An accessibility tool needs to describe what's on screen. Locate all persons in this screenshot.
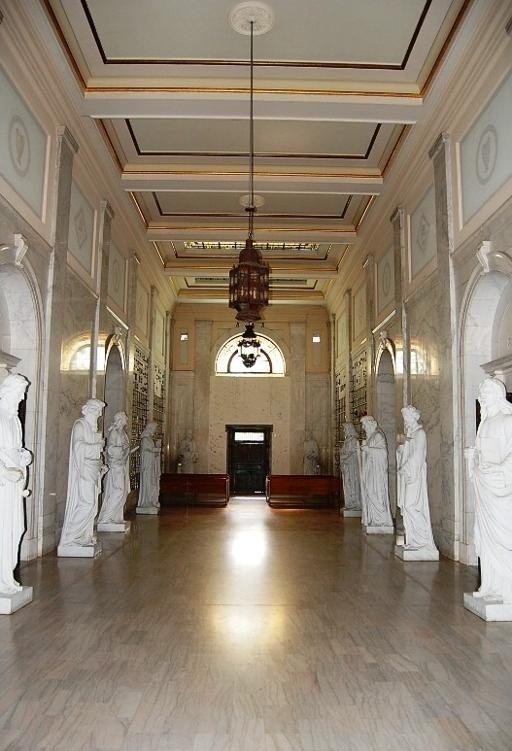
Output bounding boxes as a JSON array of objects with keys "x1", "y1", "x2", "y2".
[
  {"x1": 338, "y1": 422, "x2": 361, "y2": 509},
  {"x1": 356, "y1": 415, "x2": 394, "y2": 527},
  {"x1": 61, "y1": 397, "x2": 109, "y2": 546},
  {"x1": 98, "y1": 410, "x2": 132, "y2": 525},
  {"x1": 299, "y1": 429, "x2": 320, "y2": 475},
  {"x1": 395, "y1": 404, "x2": 434, "y2": 548},
  {"x1": 1, "y1": 374, "x2": 32, "y2": 594},
  {"x1": 137, "y1": 422, "x2": 164, "y2": 509},
  {"x1": 178, "y1": 431, "x2": 198, "y2": 473},
  {"x1": 462, "y1": 377, "x2": 512, "y2": 601}
]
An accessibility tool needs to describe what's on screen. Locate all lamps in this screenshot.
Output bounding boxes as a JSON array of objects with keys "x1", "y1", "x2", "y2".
[{"x1": 228, "y1": 1, "x2": 274, "y2": 369}]
